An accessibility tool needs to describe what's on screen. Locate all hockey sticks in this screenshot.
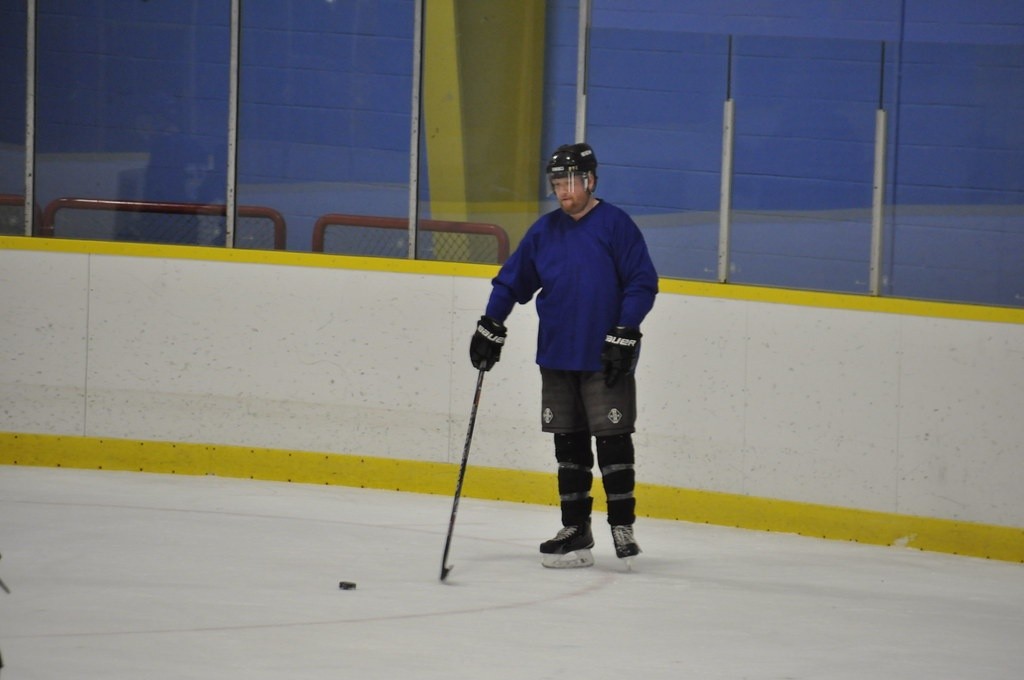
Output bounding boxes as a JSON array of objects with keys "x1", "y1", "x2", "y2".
[{"x1": 440, "y1": 359, "x2": 489, "y2": 581}]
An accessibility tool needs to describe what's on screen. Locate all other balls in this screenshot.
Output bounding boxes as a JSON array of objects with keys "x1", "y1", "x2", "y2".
[{"x1": 338, "y1": 581, "x2": 357, "y2": 591}]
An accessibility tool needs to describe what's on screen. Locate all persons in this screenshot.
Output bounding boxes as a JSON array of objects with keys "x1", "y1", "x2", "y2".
[{"x1": 470, "y1": 143, "x2": 659, "y2": 572}]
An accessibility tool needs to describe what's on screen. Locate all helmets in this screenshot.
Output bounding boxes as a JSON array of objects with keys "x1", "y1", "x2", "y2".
[{"x1": 545, "y1": 143, "x2": 599, "y2": 193}]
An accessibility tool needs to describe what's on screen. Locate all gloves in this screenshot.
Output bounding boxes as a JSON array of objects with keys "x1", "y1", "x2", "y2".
[
  {"x1": 470, "y1": 315, "x2": 508, "y2": 372},
  {"x1": 601, "y1": 323, "x2": 643, "y2": 387}
]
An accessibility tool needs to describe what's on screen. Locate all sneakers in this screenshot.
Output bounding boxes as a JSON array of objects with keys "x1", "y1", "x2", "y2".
[
  {"x1": 611, "y1": 525, "x2": 639, "y2": 572},
  {"x1": 540, "y1": 517, "x2": 595, "y2": 568}
]
